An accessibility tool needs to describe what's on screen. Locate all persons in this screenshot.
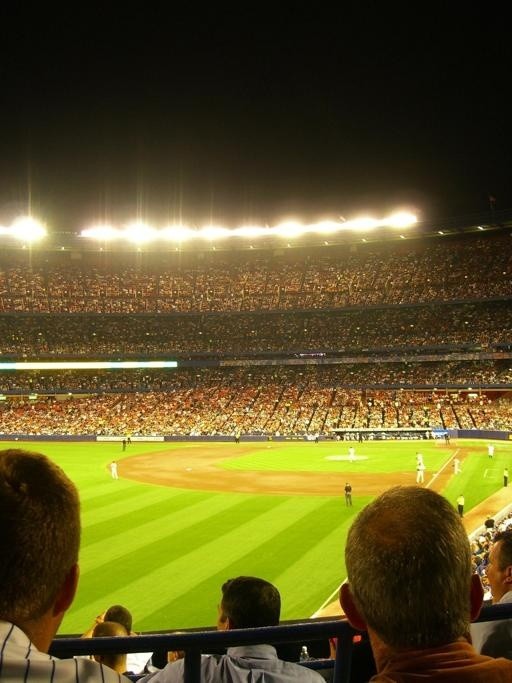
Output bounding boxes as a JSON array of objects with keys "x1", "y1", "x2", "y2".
[
  {"x1": 127, "y1": 431, "x2": 133, "y2": 443},
  {"x1": 344, "y1": 482, "x2": 352, "y2": 506},
  {"x1": 268, "y1": 433, "x2": 274, "y2": 447},
  {"x1": 235, "y1": 431, "x2": 240, "y2": 444},
  {"x1": 488, "y1": 442, "x2": 494, "y2": 458},
  {"x1": 451, "y1": 456, "x2": 462, "y2": 474},
  {"x1": 0, "y1": 446, "x2": 130, "y2": 681},
  {"x1": 349, "y1": 446, "x2": 358, "y2": 463},
  {"x1": 443, "y1": 433, "x2": 451, "y2": 446},
  {"x1": 503, "y1": 467, "x2": 508, "y2": 487},
  {"x1": 110, "y1": 459, "x2": 120, "y2": 479},
  {"x1": 69, "y1": 488, "x2": 511, "y2": 681},
  {"x1": 1, "y1": 233, "x2": 511, "y2": 435},
  {"x1": 415, "y1": 451, "x2": 425, "y2": 468},
  {"x1": 416, "y1": 461, "x2": 424, "y2": 482},
  {"x1": 122, "y1": 438, "x2": 126, "y2": 449}
]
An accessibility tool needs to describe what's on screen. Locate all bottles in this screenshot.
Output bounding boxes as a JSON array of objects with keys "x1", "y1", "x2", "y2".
[{"x1": 298, "y1": 646, "x2": 312, "y2": 661}]
